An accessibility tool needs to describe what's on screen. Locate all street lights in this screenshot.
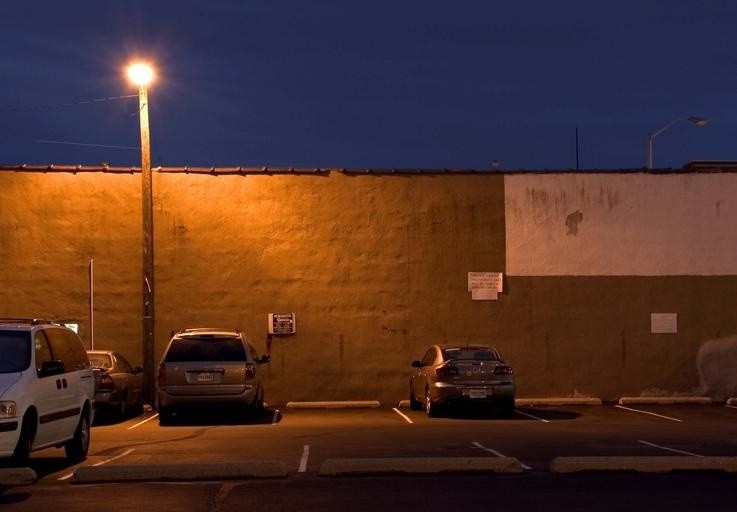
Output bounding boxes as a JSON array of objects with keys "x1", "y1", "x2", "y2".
[{"x1": 118, "y1": 55, "x2": 165, "y2": 414}]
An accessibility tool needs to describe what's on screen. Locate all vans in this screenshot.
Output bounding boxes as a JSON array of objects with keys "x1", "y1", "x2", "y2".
[{"x1": 0, "y1": 315, "x2": 97, "y2": 468}]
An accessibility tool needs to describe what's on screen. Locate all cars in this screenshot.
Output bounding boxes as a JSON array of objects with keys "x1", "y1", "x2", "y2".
[
  {"x1": 154, "y1": 326, "x2": 271, "y2": 417},
  {"x1": 408, "y1": 341, "x2": 517, "y2": 418},
  {"x1": 86, "y1": 349, "x2": 144, "y2": 421}
]
